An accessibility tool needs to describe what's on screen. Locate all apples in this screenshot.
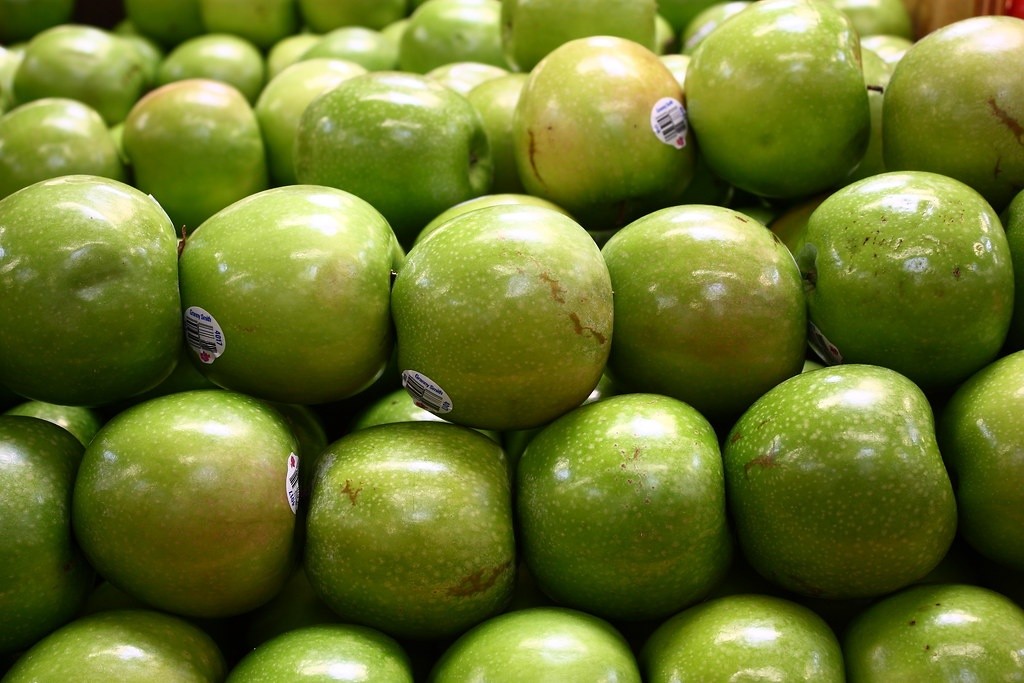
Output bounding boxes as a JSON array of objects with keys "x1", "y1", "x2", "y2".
[{"x1": 0, "y1": 0, "x2": 1024, "y2": 683}]
